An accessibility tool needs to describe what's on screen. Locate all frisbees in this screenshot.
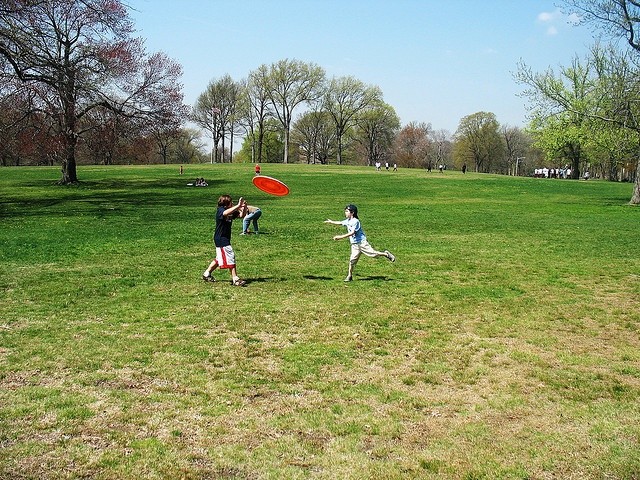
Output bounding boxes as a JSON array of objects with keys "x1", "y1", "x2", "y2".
[{"x1": 252, "y1": 176, "x2": 289, "y2": 196}]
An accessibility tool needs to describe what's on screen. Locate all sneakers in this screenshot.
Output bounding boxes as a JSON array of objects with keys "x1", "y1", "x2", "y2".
[
  {"x1": 239, "y1": 233, "x2": 246, "y2": 235},
  {"x1": 253, "y1": 231, "x2": 259, "y2": 234},
  {"x1": 384, "y1": 249, "x2": 395, "y2": 262},
  {"x1": 344, "y1": 277, "x2": 352, "y2": 282}
]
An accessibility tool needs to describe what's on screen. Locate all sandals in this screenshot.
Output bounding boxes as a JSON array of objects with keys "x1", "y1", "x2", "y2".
[
  {"x1": 232, "y1": 278, "x2": 246, "y2": 286},
  {"x1": 202, "y1": 274, "x2": 216, "y2": 282}
]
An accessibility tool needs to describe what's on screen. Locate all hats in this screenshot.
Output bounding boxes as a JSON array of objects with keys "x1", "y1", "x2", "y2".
[{"x1": 346, "y1": 204, "x2": 359, "y2": 219}]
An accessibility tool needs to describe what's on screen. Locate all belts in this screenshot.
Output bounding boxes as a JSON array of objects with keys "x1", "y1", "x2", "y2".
[{"x1": 257, "y1": 209, "x2": 261, "y2": 210}]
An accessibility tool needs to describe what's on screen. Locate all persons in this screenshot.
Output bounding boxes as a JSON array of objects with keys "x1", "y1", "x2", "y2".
[
  {"x1": 427, "y1": 160, "x2": 432, "y2": 172},
  {"x1": 534, "y1": 167, "x2": 572, "y2": 179},
  {"x1": 375, "y1": 162, "x2": 381, "y2": 171},
  {"x1": 443, "y1": 163, "x2": 446, "y2": 170},
  {"x1": 386, "y1": 162, "x2": 390, "y2": 171},
  {"x1": 203, "y1": 195, "x2": 248, "y2": 287},
  {"x1": 439, "y1": 164, "x2": 443, "y2": 172},
  {"x1": 324, "y1": 204, "x2": 396, "y2": 282},
  {"x1": 393, "y1": 163, "x2": 397, "y2": 171},
  {"x1": 196, "y1": 177, "x2": 208, "y2": 187},
  {"x1": 255, "y1": 164, "x2": 261, "y2": 176},
  {"x1": 462, "y1": 165, "x2": 466, "y2": 173},
  {"x1": 585, "y1": 171, "x2": 590, "y2": 180},
  {"x1": 232, "y1": 206, "x2": 262, "y2": 236},
  {"x1": 180, "y1": 165, "x2": 183, "y2": 174}
]
